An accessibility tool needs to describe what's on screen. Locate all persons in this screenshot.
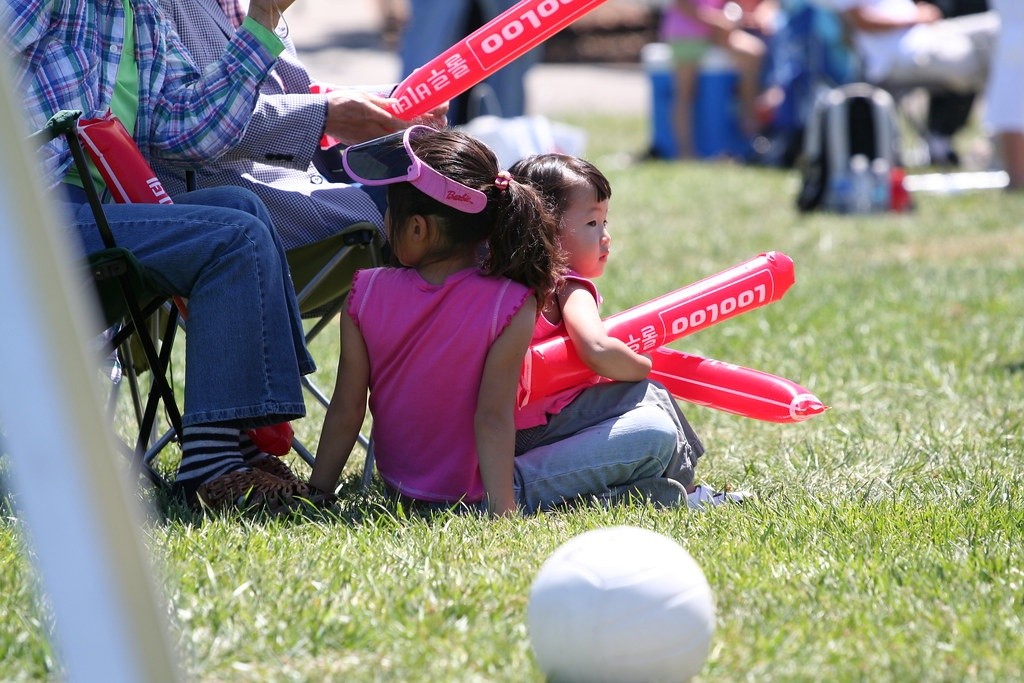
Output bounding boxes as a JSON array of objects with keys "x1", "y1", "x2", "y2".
[
  {"x1": 152, "y1": 0, "x2": 496, "y2": 319},
  {"x1": 0, "y1": 0, "x2": 333, "y2": 520},
  {"x1": 659, "y1": 0, "x2": 1023, "y2": 195},
  {"x1": 507, "y1": 153, "x2": 705, "y2": 468},
  {"x1": 402, "y1": 0, "x2": 529, "y2": 122},
  {"x1": 297, "y1": 125, "x2": 751, "y2": 520}
]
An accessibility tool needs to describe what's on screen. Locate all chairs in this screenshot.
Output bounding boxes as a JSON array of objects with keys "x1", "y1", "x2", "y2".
[{"x1": 5, "y1": 111, "x2": 380, "y2": 504}]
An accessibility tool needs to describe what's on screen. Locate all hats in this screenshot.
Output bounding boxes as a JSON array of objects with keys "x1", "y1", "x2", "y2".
[{"x1": 342, "y1": 125, "x2": 487, "y2": 212}]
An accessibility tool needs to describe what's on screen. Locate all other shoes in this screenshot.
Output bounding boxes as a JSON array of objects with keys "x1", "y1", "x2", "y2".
[
  {"x1": 597, "y1": 477, "x2": 688, "y2": 508},
  {"x1": 194, "y1": 455, "x2": 334, "y2": 518}
]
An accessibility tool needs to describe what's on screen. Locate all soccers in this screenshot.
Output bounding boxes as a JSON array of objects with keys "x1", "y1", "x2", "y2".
[{"x1": 522, "y1": 521, "x2": 721, "y2": 683}]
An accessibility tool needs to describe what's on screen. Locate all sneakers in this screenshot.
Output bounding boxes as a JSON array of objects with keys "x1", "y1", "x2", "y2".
[{"x1": 684, "y1": 485, "x2": 743, "y2": 511}]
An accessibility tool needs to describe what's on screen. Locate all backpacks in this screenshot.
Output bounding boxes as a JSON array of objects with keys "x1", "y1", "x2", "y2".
[{"x1": 797, "y1": 83, "x2": 896, "y2": 213}]
